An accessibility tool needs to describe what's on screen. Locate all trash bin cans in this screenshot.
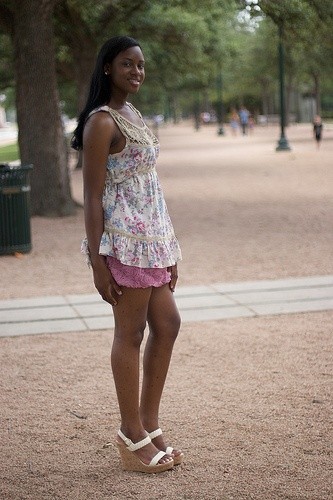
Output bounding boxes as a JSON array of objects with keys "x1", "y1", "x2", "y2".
[{"x1": 0, "y1": 163, "x2": 33, "y2": 255}]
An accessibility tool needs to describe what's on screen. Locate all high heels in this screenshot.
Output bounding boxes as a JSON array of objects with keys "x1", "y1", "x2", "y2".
[
  {"x1": 115, "y1": 429, "x2": 174, "y2": 472},
  {"x1": 145, "y1": 428, "x2": 185, "y2": 464}
]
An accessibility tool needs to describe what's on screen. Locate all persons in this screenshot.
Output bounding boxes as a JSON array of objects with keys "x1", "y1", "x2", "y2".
[
  {"x1": 312, "y1": 116, "x2": 323, "y2": 147},
  {"x1": 229, "y1": 107, "x2": 239, "y2": 133},
  {"x1": 240, "y1": 106, "x2": 249, "y2": 135},
  {"x1": 70, "y1": 36, "x2": 184, "y2": 471}
]
